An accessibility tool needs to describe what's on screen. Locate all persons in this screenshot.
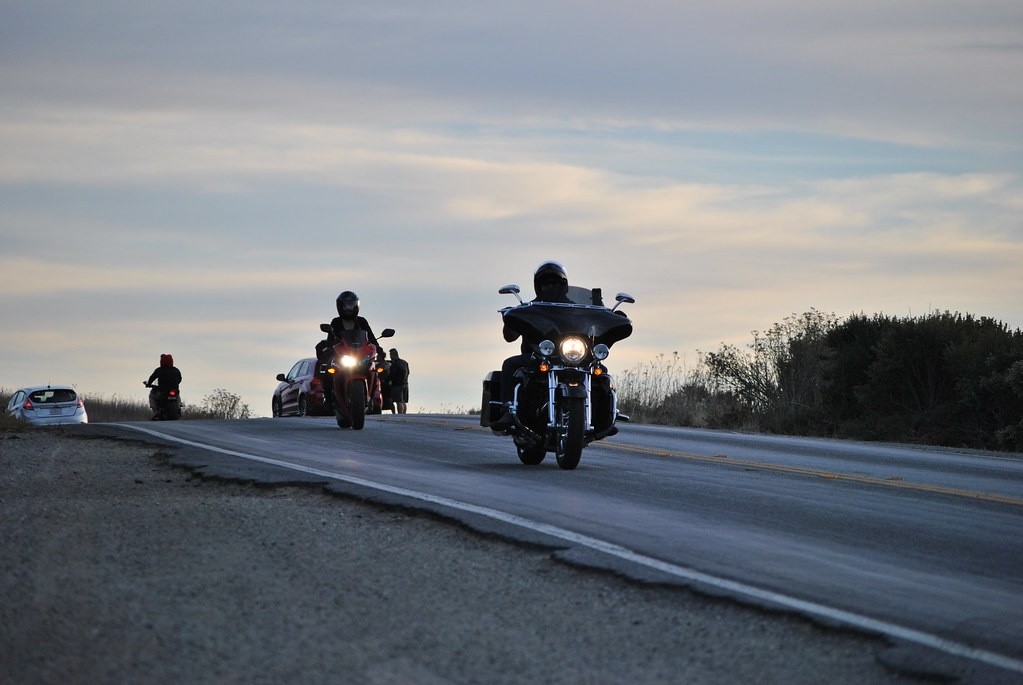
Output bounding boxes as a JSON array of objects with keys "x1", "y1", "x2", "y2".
[
  {"x1": 375, "y1": 347, "x2": 410, "y2": 414},
  {"x1": 323, "y1": 291, "x2": 386, "y2": 409},
  {"x1": 491, "y1": 262, "x2": 619, "y2": 439},
  {"x1": 146, "y1": 354, "x2": 182, "y2": 420}
]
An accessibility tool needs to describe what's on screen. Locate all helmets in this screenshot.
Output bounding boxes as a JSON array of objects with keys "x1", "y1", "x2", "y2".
[
  {"x1": 336, "y1": 291, "x2": 360, "y2": 320},
  {"x1": 533, "y1": 262, "x2": 568, "y2": 301}
]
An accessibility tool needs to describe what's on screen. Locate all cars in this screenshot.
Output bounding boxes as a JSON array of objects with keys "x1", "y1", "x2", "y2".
[
  {"x1": 271, "y1": 357, "x2": 383, "y2": 418},
  {"x1": 5, "y1": 385, "x2": 88, "y2": 425}
]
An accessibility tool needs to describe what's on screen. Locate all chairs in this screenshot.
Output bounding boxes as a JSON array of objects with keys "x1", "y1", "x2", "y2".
[
  {"x1": 45, "y1": 397, "x2": 53, "y2": 402},
  {"x1": 35, "y1": 398, "x2": 41, "y2": 402}
]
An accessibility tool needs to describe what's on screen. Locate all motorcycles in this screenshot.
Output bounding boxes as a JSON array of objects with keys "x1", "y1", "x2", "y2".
[
  {"x1": 479, "y1": 285, "x2": 636, "y2": 470},
  {"x1": 315, "y1": 324, "x2": 395, "y2": 430}
]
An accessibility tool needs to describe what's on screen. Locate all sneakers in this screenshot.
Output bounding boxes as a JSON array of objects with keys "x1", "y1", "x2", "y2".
[
  {"x1": 606, "y1": 426, "x2": 618, "y2": 437},
  {"x1": 491, "y1": 412, "x2": 514, "y2": 430}
]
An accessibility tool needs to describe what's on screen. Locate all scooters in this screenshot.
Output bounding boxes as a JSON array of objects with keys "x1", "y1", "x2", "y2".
[{"x1": 144, "y1": 381, "x2": 185, "y2": 420}]
